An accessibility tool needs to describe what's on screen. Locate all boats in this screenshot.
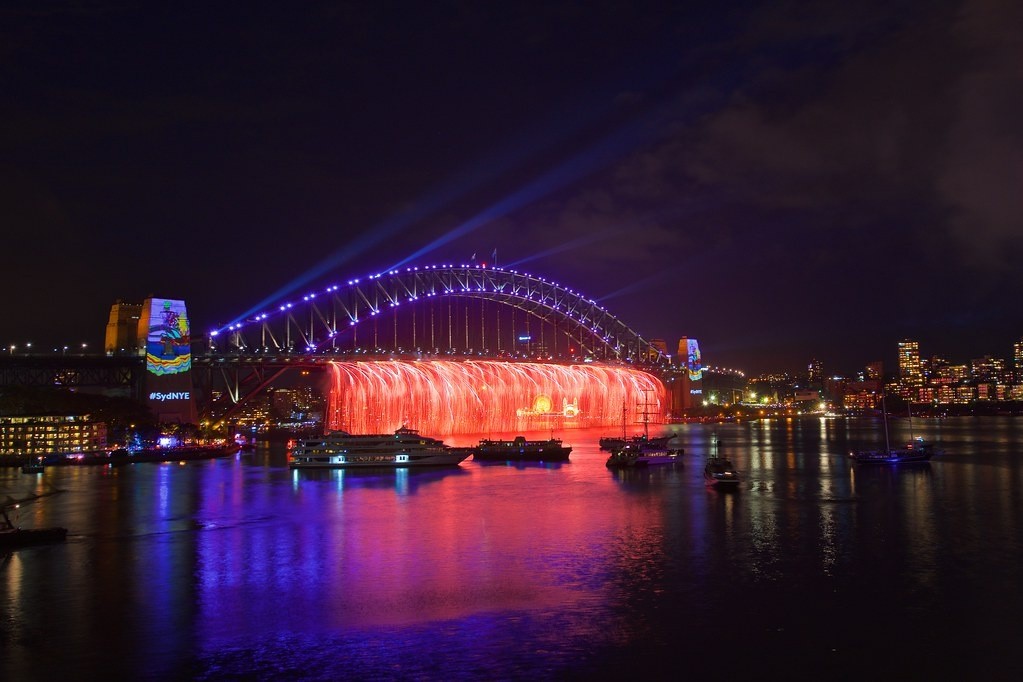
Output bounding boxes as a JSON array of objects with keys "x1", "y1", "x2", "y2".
[
  {"x1": 22, "y1": 451, "x2": 44, "y2": 474},
  {"x1": 704, "y1": 427, "x2": 742, "y2": 486},
  {"x1": 471, "y1": 437, "x2": 573, "y2": 462},
  {"x1": 285, "y1": 428, "x2": 476, "y2": 469}
]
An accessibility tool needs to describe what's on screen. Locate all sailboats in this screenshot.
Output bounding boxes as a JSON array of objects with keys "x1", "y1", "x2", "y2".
[
  {"x1": 852, "y1": 385, "x2": 944, "y2": 463},
  {"x1": 599, "y1": 397, "x2": 678, "y2": 449},
  {"x1": 607, "y1": 388, "x2": 689, "y2": 467}
]
